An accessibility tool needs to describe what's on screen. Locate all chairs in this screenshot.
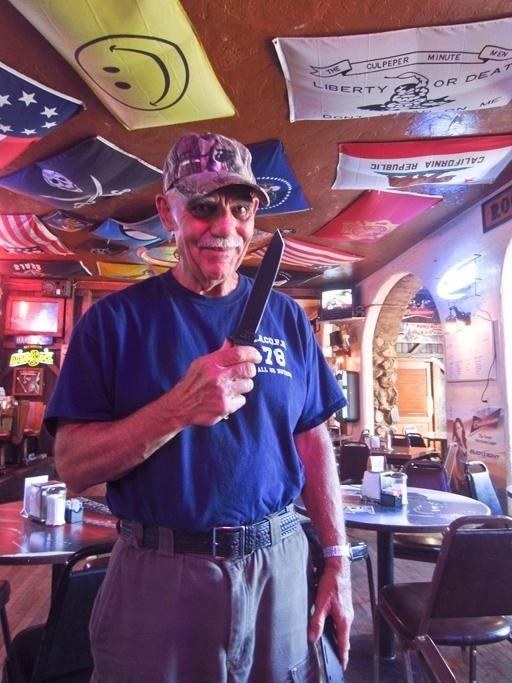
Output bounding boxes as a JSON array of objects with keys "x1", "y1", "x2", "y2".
[{"x1": 0, "y1": 541, "x2": 116, "y2": 682}]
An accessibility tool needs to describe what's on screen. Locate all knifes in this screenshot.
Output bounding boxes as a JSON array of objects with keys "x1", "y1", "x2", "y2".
[{"x1": 224, "y1": 227, "x2": 287, "y2": 349}]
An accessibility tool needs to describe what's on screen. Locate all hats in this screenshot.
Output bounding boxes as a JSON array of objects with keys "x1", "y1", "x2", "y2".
[{"x1": 161, "y1": 132, "x2": 272, "y2": 212}]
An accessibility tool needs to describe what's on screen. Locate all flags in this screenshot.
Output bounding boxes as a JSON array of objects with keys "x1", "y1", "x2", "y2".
[
  {"x1": 0, "y1": 206, "x2": 277, "y2": 279},
  {"x1": 0, "y1": 134, "x2": 164, "y2": 211},
  {"x1": 331, "y1": 132, "x2": 512, "y2": 190},
  {"x1": 0, "y1": 58, "x2": 88, "y2": 176},
  {"x1": 249, "y1": 237, "x2": 369, "y2": 269},
  {"x1": 8, "y1": 0, "x2": 244, "y2": 133},
  {"x1": 272, "y1": 16, "x2": 512, "y2": 125},
  {"x1": 242, "y1": 138, "x2": 312, "y2": 218},
  {"x1": 307, "y1": 188, "x2": 444, "y2": 246},
  {"x1": 272, "y1": 271, "x2": 324, "y2": 287}
]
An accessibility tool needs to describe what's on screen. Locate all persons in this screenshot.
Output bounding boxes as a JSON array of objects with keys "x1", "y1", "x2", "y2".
[
  {"x1": 44, "y1": 132, "x2": 354, "y2": 682},
  {"x1": 451, "y1": 417, "x2": 468, "y2": 491}
]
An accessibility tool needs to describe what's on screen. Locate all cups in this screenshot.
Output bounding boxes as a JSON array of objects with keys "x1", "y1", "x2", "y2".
[{"x1": 370, "y1": 435, "x2": 380, "y2": 450}]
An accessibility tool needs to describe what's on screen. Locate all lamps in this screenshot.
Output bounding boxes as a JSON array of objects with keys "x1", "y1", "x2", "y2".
[{"x1": 445, "y1": 305, "x2": 471, "y2": 327}]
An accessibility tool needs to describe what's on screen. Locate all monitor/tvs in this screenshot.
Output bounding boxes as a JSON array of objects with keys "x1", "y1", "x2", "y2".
[
  {"x1": 4, "y1": 296, "x2": 64, "y2": 337},
  {"x1": 319, "y1": 283, "x2": 357, "y2": 318}
]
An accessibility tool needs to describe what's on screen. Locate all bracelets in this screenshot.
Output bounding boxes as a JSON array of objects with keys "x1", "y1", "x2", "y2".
[{"x1": 321, "y1": 541, "x2": 351, "y2": 559}]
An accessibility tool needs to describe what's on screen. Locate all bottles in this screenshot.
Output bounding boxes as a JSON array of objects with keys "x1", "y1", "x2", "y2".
[
  {"x1": 45, "y1": 486, "x2": 65, "y2": 525},
  {"x1": 384, "y1": 432, "x2": 392, "y2": 449},
  {"x1": 391, "y1": 471, "x2": 408, "y2": 506}
]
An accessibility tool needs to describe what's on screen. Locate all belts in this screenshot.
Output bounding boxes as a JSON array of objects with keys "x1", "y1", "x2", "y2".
[{"x1": 120, "y1": 505, "x2": 299, "y2": 560}]
